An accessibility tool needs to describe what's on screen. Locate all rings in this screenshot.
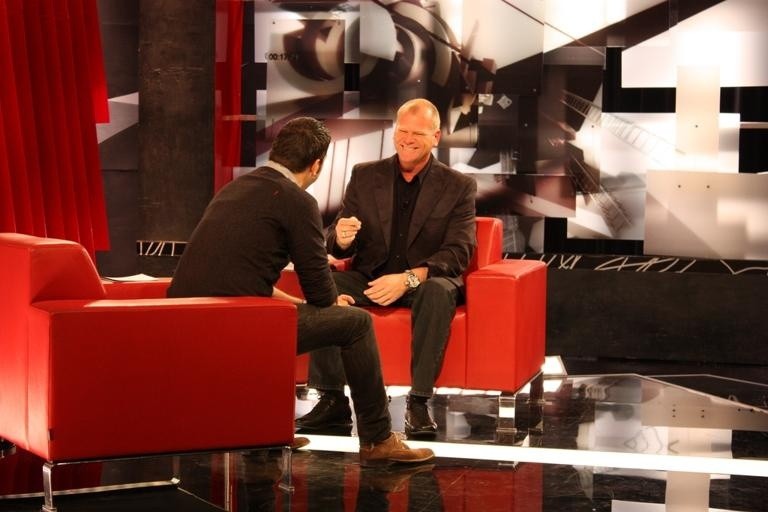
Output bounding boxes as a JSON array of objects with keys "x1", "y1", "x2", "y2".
[{"x1": 342, "y1": 231, "x2": 346, "y2": 238}]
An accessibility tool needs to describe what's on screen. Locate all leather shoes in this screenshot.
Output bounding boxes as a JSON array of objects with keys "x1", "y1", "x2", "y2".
[
  {"x1": 360, "y1": 431, "x2": 435, "y2": 465},
  {"x1": 295, "y1": 396, "x2": 354, "y2": 436},
  {"x1": 294, "y1": 436, "x2": 310, "y2": 450},
  {"x1": 404, "y1": 396, "x2": 438, "y2": 436}
]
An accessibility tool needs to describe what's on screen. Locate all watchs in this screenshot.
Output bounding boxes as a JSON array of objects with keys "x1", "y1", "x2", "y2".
[{"x1": 404, "y1": 269, "x2": 421, "y2": 295}]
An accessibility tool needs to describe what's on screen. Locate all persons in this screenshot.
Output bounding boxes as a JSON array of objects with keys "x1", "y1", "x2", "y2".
[
  {"x1": 165, "y1": 114, "x2": 437, "y2": 468},
  {"x1": 294, "y1": 96, "x2": 480, "y2": 435}
]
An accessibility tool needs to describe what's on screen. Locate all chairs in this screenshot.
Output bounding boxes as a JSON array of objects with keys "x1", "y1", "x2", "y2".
[
  {"x1": 1, "y1": 232, "x2": 299, "y2": 512},
  {"x1": 273, "y1": 215, "x2": 547, "y2": 435}
]
